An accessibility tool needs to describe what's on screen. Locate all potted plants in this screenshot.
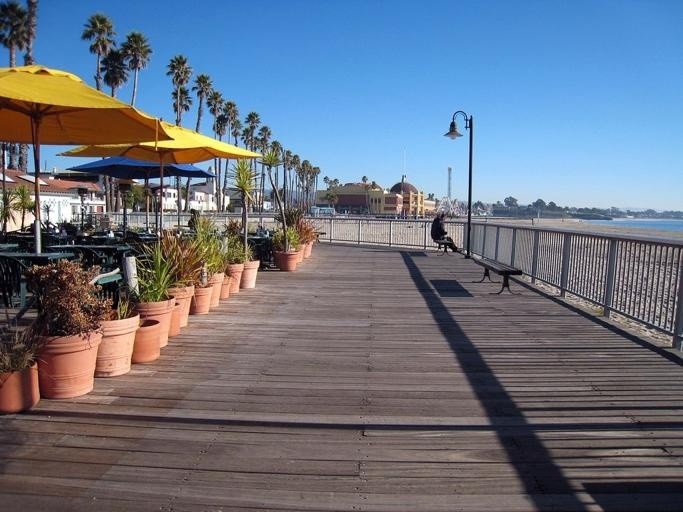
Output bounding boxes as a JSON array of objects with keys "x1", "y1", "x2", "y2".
[
  {"x1": 0, "y1": 315, "x2": 53, "y2": 413},
  {"x1": 92, "y1": 296, "x2": 140, "y2": 378},
  {"x1": 24, "y1": 256, "x2": 115, "y2": 398},
  {"x1": 136, "y1": 207, "x2": 317, "y2": 346}
]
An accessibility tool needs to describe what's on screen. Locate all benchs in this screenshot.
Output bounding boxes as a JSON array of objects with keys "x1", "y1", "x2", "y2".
[
  {"x1": 429, "y1": 236, "x2": 455, "y2": 256},
  {"x1": 470, "y1": 255, "x2": 522, "y2": 296}
]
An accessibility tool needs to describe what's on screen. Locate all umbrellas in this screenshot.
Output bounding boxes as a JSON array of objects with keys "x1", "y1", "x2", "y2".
[
  {"x1": 0, "y1": 60, "x2": 171, "y2": 258},
  {"x1": 63, "y1": 155, "x2": 213, "y2": 231},
  {"x1": 52, "y1": 116, "x2": 260, "y2": 233}
]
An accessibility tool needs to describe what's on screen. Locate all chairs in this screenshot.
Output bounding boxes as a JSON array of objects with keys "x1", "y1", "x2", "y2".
[{"x1": 1, "y1": 219, "x2": 272, "y2": 309}]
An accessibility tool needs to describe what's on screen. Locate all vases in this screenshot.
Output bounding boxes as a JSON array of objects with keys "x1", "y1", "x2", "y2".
[{"x1": 131, "y1": 318, "x2": 160, "y2": 364}]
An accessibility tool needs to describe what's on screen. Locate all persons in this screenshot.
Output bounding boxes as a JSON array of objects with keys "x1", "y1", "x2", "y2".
[{"x1": 430, "y1": 210, "x2": 464, "y2": 253}]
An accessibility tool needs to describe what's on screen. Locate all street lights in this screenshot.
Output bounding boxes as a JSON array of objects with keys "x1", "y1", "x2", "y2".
[{"x1": 443, "y1": 110, "x2": 474, "y2": 259}]
